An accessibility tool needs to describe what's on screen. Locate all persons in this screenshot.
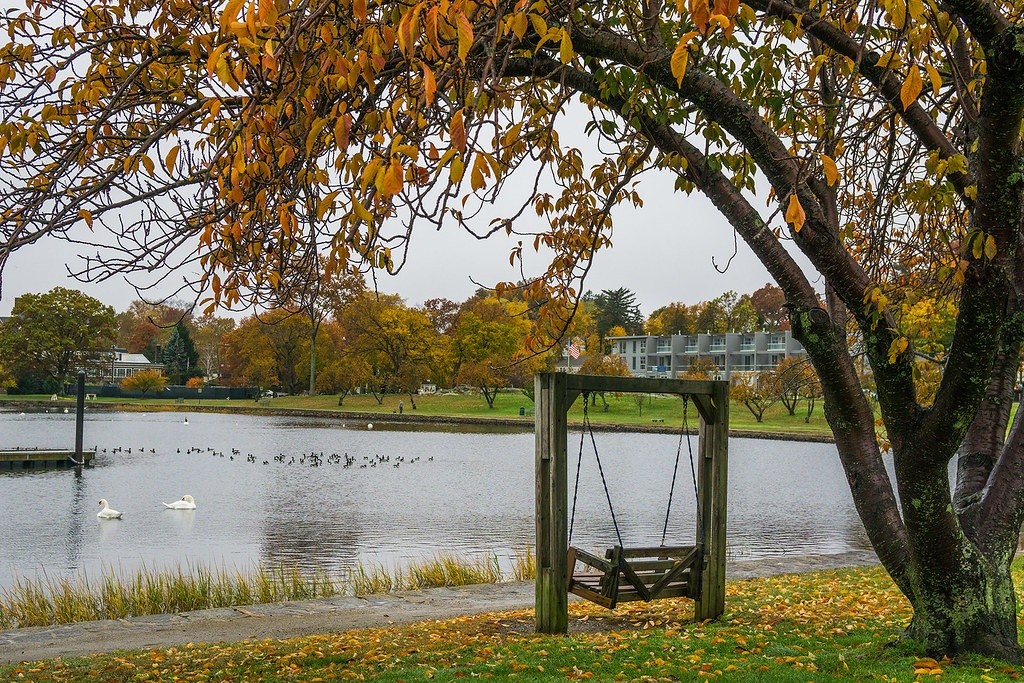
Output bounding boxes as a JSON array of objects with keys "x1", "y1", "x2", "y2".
[{"x1": 399, "y1": 400, "x2": 403, "y2": 413}]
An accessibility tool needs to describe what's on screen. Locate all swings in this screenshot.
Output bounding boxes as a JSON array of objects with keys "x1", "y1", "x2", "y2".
[{"x1": 567, "y1": 394, "x2": 706, "y2": 608}]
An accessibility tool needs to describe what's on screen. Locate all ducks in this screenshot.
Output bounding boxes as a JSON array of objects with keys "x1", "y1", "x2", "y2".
[
  {"x1": 163, "y1": 495, "x2": 196, "y2": 509},
  {"x1": 96, "y1": 499, "x2": 125, "y2": 519},
  {"x1": 89, "y1": 445, "x2": 436, "y2": 470}
]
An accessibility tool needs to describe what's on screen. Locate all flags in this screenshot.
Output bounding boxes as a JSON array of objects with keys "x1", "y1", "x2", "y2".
[{"x1": 569, "y1": 339, "x2": 580, "y2": 360}]
